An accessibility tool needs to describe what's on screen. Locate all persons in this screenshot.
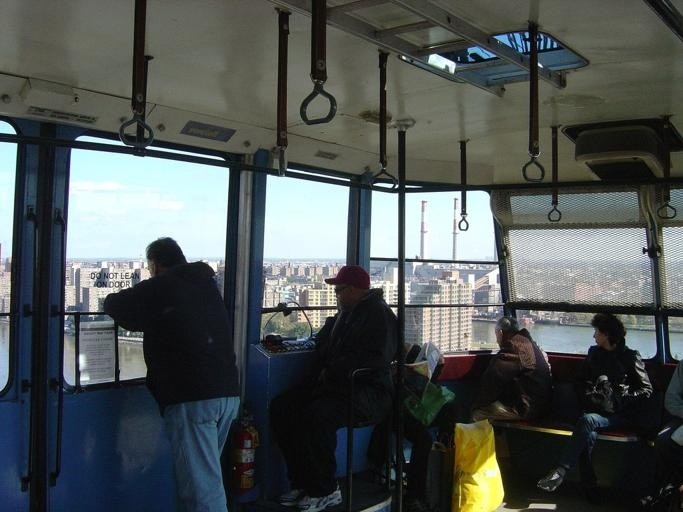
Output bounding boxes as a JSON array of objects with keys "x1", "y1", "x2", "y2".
[
  {"x1": 468, "y1": 315, "x2": 553, "y2": 479},
  {"x1": 266, "y1": 264, "x2": 402, "y2": 511},
  {"x1": 652, "y1": 356, "x2": 683, "y2": 500},
  {"x1": 104, "y1": 235, "x2": 242, "y2": 510},
  {"x1": 527, "y1": 307, "x2": 654, "y2": 501}
]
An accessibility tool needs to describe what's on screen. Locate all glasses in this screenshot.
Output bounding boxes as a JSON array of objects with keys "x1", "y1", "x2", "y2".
[{"x1": 334, "y1": 286, "x2": 348, "y2": 294}]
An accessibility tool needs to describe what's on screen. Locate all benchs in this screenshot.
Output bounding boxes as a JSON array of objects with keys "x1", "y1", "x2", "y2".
[{"x1": 468, "y1": 354, "x2": 676, "y2": 444}]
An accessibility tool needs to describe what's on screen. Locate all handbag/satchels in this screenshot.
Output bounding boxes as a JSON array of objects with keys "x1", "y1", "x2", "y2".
[
  {"x1": 580, "y1": 380, "x2": 630, "y2": 415},
  {"x1": 424, "y1": 441, "x2": 455, "y2": 512}
]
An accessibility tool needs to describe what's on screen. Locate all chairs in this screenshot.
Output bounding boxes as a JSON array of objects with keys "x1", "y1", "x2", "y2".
[{"x1": 343, "y1": 348, "x2": 446, "y2": 511}]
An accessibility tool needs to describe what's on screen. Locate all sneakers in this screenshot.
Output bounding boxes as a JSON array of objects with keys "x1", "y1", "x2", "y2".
[
  {"x1": 278, "y1": 479, "x2": 343, "y2": 512},
  {"x1": 537, "y1": 470, "x2": 564, "y2": 492}
]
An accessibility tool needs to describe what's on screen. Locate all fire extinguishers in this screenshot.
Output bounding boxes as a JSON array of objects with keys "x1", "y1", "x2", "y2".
[{"x1": 233, "y1": 420, "x2": 258, "y2": 498}]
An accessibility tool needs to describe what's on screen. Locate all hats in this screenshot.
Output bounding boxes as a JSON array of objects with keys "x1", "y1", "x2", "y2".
[{"x1": 324, "y1": 266, "x2": 370, "y2": 290}]
